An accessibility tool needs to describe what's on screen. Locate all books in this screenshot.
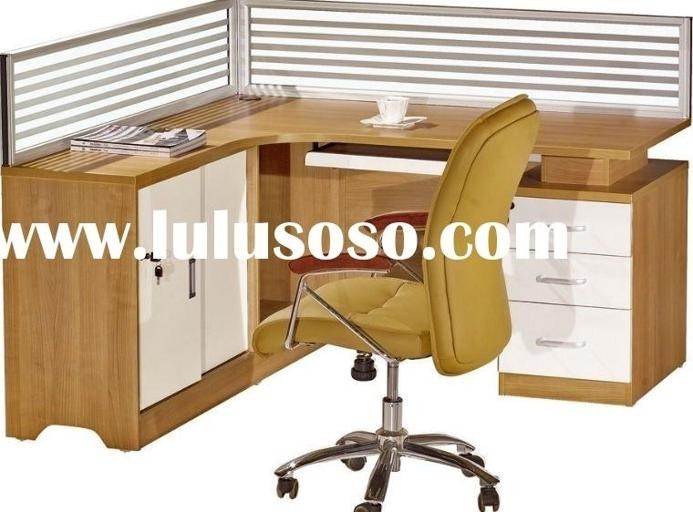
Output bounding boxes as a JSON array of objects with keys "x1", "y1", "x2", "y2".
[{"x1": 70, "y1": 124, "x2": 208, "y2": 158}]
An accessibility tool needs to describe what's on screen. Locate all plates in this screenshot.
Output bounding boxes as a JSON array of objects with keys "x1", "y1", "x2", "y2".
[{"x1": 360, "y1": 113, "x2": 427, "y2": 130}]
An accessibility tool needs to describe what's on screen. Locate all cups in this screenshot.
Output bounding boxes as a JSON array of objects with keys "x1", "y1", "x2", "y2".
[{"x1": 376, "y1": 96, "x2": 409, "y2": 123}]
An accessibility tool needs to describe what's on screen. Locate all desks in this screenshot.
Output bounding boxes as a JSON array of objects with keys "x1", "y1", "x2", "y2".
[{"x1": 4, "y1": 100, "x2": 689, "y2": 452}]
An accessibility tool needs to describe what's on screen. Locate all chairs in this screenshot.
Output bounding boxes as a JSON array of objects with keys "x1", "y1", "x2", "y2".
[{"x1": 250, "y1": 93, "x2": 543, "y2": 509}]
